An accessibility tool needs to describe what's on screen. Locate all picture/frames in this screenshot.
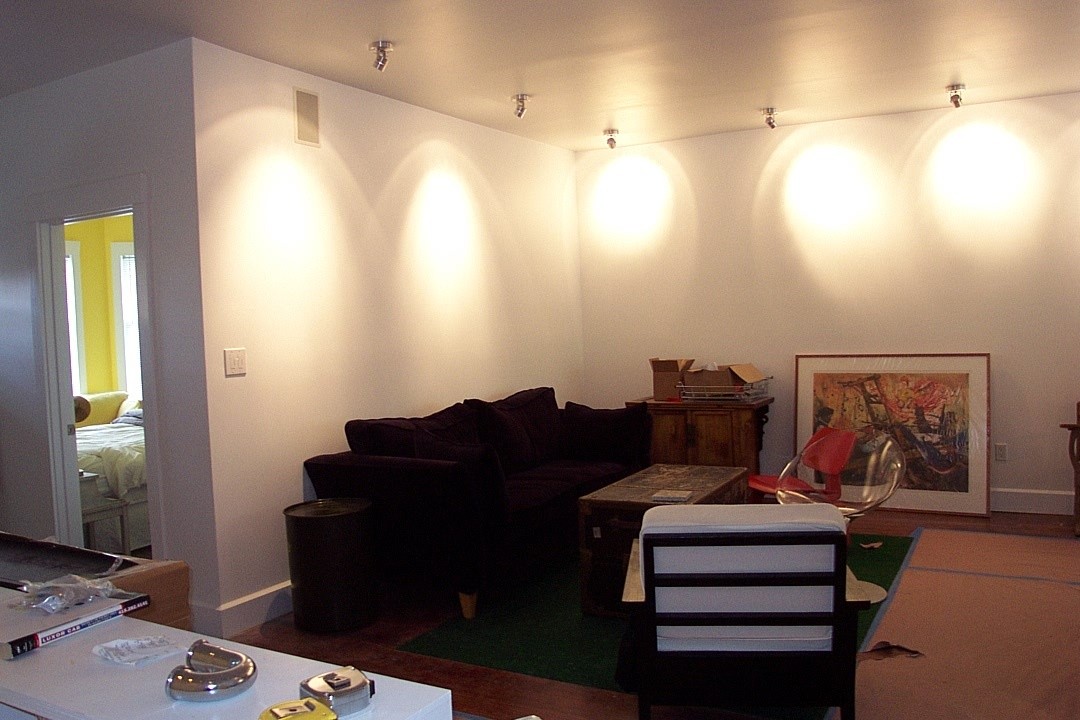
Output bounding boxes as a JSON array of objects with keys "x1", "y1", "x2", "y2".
[{"x1": 794, "y1": 355, "x2": 989, "y2": 518}]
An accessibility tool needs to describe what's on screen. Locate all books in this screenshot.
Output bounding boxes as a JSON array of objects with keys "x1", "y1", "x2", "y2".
[{"x1": 0, "y1": 582, "x2": 150, "y2": 661}]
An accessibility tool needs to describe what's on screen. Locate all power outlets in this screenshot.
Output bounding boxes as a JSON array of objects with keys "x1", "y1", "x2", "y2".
[{"x1": 995, "y1": 441, "x2": 1010, "y2": 461}]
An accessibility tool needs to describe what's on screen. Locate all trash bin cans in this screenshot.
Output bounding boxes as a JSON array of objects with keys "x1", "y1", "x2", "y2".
[{"x1": 283, "y1": 496, "x2": 376, "y2": 635}]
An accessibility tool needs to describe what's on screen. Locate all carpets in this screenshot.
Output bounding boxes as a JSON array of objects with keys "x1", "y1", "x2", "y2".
[
  {"x1": 823, "y1": 526, "x2": 1078, "y2": 720},
  {"x1": 399, "y1": 521, "x2": 925, "y2": 700}
]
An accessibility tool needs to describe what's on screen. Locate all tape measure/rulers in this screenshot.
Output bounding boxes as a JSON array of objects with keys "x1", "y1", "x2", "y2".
[
  {"x1": 297, "y1": 665, "x2": 376, "y2": 716},
  {"x1": 256, "y1": 696, "x2": 339, "y2": 720}
]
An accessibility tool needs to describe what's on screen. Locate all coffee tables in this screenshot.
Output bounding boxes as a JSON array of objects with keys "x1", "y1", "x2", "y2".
[{"x1": 578, "y1": 460, "x2": 753, "y2": 617}]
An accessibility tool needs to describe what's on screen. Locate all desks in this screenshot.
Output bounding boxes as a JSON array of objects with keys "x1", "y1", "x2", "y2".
[{"x1": 0, "y1": 583, "x2": 453, "y2": 719}]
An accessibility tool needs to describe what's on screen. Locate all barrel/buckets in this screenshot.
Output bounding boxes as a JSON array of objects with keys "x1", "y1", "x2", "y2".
[{"x1": 281, "y1": 496, "x2": 377, "y2": 632}]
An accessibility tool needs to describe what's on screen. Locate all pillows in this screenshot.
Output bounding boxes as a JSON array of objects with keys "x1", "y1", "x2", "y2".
[
  {"x1": 462, "y1": 388, "x2": 567, "y2": 475},
  {"x1": 344, "y1": 403, "x2": 505, "y2": 484}
]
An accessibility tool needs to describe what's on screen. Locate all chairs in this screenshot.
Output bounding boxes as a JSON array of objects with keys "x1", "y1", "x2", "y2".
[
  {"x1": 776, "y1": 428, "x2": 907, "y2": 603},
  {"x1": 620, "y1": 500, "x2": 873, "y2": 717},
  {"x1": 749, "y1": 425, "x2": 855, "y2": 503}
]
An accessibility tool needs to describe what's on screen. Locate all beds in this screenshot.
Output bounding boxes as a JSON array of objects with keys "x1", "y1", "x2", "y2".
[{"x1": 75, "y1": 395, "x2": 151, "y2": 553}]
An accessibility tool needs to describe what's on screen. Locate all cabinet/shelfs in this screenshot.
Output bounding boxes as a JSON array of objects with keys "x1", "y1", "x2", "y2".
[{"x1": 629, "y1": 394, "x2": 777, "y2": 508}]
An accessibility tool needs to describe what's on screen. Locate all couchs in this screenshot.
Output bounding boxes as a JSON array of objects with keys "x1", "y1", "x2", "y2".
[{"x1": 304, "y1": 409, "x2": 642, "y2": 620}]
